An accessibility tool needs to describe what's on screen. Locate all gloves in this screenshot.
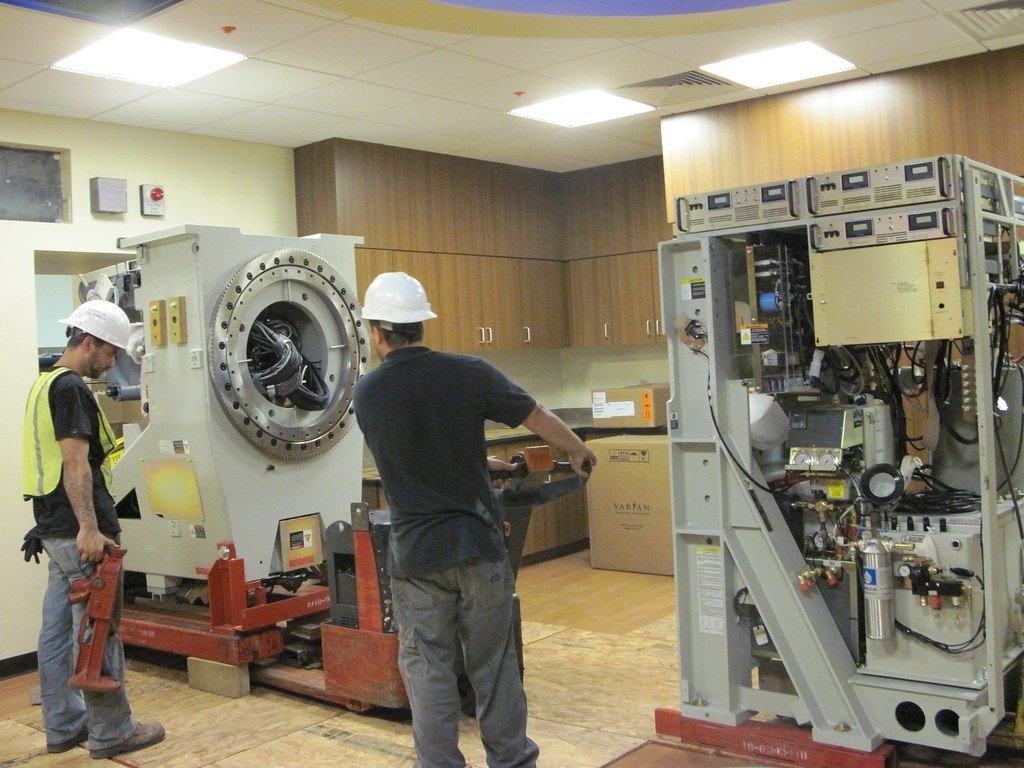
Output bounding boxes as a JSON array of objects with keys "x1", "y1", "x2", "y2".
[{"x1": 20, "y1": 533, "x2": 43, "y2": 564}]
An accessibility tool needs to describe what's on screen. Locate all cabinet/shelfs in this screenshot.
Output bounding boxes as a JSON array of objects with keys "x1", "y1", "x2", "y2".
[
  {"x1": 363, "y1": 419, "x2": 590, "y2": 570},
  {"x1": 293, "y1": 138, "x2": 673, "y2": 353}
]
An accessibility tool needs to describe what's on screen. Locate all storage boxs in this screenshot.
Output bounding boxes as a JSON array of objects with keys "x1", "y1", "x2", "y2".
[
  {"x1": 584, "y1": 433, "x2": 675, "y2": 577},
  {"x1": 590, "y1": 383, "x2": 670, "y2": 429}
]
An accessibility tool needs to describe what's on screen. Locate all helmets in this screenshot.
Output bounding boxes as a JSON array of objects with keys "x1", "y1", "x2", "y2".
[
  {"x1": 58, "y1": 300, "x2": 131, "y2": 350},
  {"x1": 361, "y1": 271, "x2": 437, "y2": 324}
]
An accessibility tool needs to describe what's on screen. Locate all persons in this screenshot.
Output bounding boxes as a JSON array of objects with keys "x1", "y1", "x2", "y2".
[
  {"x1": 22, "y1": 300, "x2": 166, "y2": 758},
  {"x1": 352, "y1": 272, "x2": 596, "y2": 768}
]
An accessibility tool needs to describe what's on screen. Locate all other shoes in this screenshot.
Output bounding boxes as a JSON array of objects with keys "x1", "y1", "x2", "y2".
[
  {"x1": 47, "y1": 726, "x2": 89, "y2": 753},
  {"x1": 90, "y1": 721, "x2": 165, "y2": 759}
]
